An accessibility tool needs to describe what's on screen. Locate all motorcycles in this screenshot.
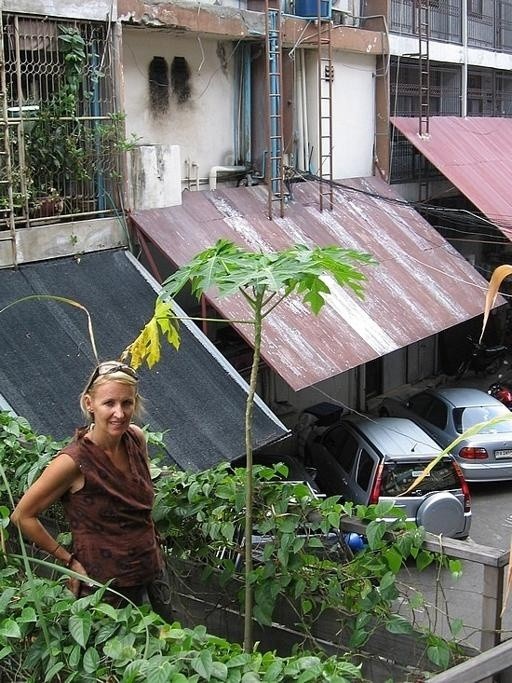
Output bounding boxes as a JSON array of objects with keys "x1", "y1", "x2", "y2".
[{"x1": 453, "y1": 332, "x2": 511, "y2": 385}]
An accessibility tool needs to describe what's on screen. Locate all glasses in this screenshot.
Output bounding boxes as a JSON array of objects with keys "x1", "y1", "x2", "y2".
[{"x1": 83, "y1": 364, "x2": 141, "y2": 397}]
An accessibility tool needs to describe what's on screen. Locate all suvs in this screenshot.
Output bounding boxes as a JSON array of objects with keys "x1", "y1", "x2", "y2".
[
  {"x1": 224, "y1": 453, "x2": 350, "y2": 580},
  {"x1": 304, "y1": 413, "x2": 475, "y2": 542}
]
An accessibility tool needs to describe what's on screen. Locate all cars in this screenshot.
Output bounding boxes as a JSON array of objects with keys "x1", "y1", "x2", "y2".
[
  {"x1": 376, "y1": 384, "x2": 512, "y2": 487},
  {"x1": 487, "y1": 369, "x2": 512, "y2": 408}
]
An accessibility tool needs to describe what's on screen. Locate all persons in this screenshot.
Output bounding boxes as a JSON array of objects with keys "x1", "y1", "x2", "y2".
[{"x1": 10, "y1": 359, "x2": 175, "y2": 627}]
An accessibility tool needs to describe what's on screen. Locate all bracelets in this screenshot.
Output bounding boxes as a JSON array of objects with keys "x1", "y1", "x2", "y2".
[
  {"x1": 61, "y1": 551, "x2": 76, "y2": 569},
  {"x1": 49, "y1": 541, "x2": 63, "y2": 555}
]
